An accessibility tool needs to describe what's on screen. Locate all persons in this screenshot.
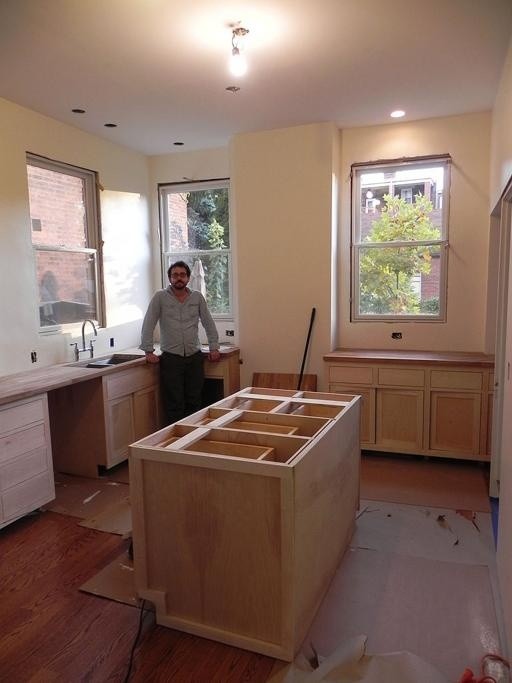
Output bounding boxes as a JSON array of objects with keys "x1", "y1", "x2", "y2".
[{"x1": 134, "y1": 258, "x2": 220, "y2": 428}]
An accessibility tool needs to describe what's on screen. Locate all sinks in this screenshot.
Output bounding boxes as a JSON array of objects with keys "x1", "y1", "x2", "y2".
[{"x1": 61, "y1": 353, "x2": 146, "y2": 369}]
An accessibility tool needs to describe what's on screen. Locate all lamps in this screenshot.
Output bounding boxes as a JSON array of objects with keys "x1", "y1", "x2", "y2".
[
  {"x1": 366, "y1": 189, "x2": 373, "y2": 199},
  {"x1": 223, "y1": 20, "x2": 250, "y2": 97}
]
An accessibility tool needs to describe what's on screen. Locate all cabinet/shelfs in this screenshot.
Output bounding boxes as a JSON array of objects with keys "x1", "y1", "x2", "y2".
[
  {"x1": 204, "y1": 348, "x2": 241, "y2": 397},
  {"x1": 0, "y1": 391, "x2": 56, "y2": 530},
  {"x1": 426, "y1": 360, "x2": 494, "y2": 461},
  {"x1": 327, "y1": 361, "x2": 426, "y2": 456},
  {"x1": 128, "y1": 388, "x2": 361, "y2": 664},
  {"x1": 48, "y1": 359, "x2": 159, "y2": 470}
]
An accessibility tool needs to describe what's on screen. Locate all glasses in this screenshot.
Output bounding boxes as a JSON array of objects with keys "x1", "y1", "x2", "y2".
[{"x1": 170, "y1": 272, "x2": 187, "y2": 279}]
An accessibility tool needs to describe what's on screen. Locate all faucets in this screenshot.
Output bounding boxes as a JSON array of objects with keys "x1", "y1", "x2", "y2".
[{"x1": 82, "y1": 318, "x2": 97, "y2": 350}]
